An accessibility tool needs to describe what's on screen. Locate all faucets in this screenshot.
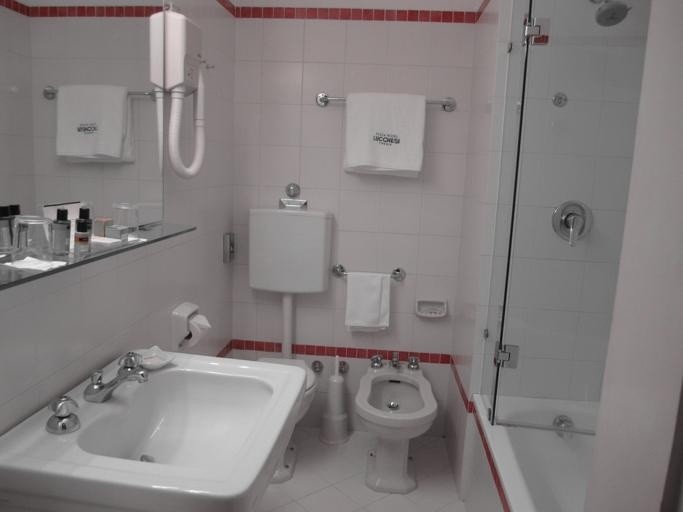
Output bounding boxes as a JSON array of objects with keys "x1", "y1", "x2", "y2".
[
  {"x1": 83, "y1": 364, "x2": 149, "y2": 404},
  {"x1": 550, "y1": 200, "x2": 593, "y2": 247},
  {"x1": 389, "y1": 351, "x2": 402, "y2": 368}
]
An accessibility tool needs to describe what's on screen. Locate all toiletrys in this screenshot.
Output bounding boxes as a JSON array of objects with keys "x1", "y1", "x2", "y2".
[{"x1": 48, "y1": 208, "x2": 92, "y2": 254}]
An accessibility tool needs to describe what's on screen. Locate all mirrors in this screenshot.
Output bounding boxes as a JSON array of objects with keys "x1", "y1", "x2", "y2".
[{"x1": 0, "y1": 1, "x2": 196, "y2": 291}]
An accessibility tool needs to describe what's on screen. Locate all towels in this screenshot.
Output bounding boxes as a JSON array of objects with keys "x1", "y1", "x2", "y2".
[
  {"x1": 344, "y1": 272, "x2": 390, "y2": 335},
  {"x1": 342, "y1": 91, "x2": 426, "y2": 180},
  {"x1": 56, "y1": 84, "x2": 135, "y2": 166}
]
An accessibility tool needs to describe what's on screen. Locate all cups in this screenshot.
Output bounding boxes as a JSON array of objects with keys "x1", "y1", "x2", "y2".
[
  {"x1": 112, "y1": 202, "x2": 140, "y2": 244},
  {"x1": 11, "y1": 215, "x2": 54, "y2": 271}
]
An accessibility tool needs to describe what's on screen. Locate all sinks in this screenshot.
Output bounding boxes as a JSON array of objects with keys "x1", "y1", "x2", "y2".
[
  {"x1": 0, "y1": 347, "x2": 306, "y2": 512},
  {"x1": 354, "y1": 361, "x2": 439, "y2": 493}
]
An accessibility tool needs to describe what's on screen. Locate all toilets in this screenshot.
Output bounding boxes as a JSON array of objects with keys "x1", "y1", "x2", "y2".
[{"x1": 247, "y1": 207, "x2": 334, "y2": 483}]
